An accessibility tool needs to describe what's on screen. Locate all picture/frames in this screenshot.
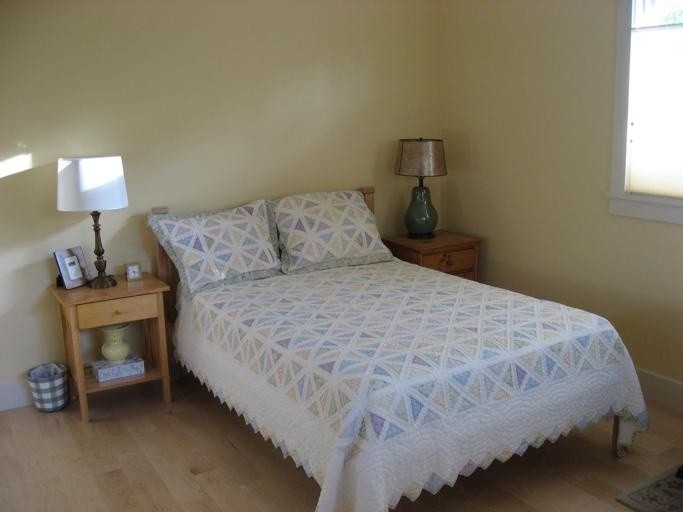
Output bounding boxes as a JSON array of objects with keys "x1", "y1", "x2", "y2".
[{"x1": 53, "y1": 245, "x2": 92, "y2": 290}]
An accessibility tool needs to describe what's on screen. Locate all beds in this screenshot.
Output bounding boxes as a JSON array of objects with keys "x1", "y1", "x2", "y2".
[{"x1": 152, "y1": 186, "x2": 621, "y2": 511}]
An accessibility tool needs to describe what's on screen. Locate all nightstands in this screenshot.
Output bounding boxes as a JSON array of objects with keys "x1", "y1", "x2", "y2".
[
  {"x1": 382, "y1": 229, "x2": 480, "y2": 280},
  {"x1": 49, "y1": 271, "x2": 172, "y2": 422}
]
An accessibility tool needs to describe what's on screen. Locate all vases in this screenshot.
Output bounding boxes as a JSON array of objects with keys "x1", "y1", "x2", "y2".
[{"x1": 100, "y1": 323, "x2": 133, "y2": 365}]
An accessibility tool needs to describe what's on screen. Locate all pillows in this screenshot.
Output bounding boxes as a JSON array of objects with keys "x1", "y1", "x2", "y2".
[
  {"x1": 271, "y1": 190, "x2": 394, "y2": 274},
  {"x1": 146, "y1": 199, "x2": 284, "y2": 298}
]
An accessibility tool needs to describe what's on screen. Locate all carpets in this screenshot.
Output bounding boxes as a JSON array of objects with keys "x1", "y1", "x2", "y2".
[{"x1": 615, "y1": 463, "x2": 683, "y2": 512}]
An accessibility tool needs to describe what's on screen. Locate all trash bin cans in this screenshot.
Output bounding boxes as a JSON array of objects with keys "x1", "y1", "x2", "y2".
[{"x1": 24, "y1": 362, "x2": 68, "y2": 412}]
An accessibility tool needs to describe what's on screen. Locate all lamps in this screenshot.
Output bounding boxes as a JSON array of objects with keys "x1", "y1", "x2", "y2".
[
  {"x1": 56, "y1": 155, "x2": 128, "y2": 289},
  {"x1": 394, "y1": 137, "x2": 447, "y2": 239}
]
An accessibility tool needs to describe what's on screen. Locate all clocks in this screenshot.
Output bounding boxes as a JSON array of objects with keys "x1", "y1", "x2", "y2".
[{"x1": 125, "y1": 262, "x2": 142, "y2": 282}]
